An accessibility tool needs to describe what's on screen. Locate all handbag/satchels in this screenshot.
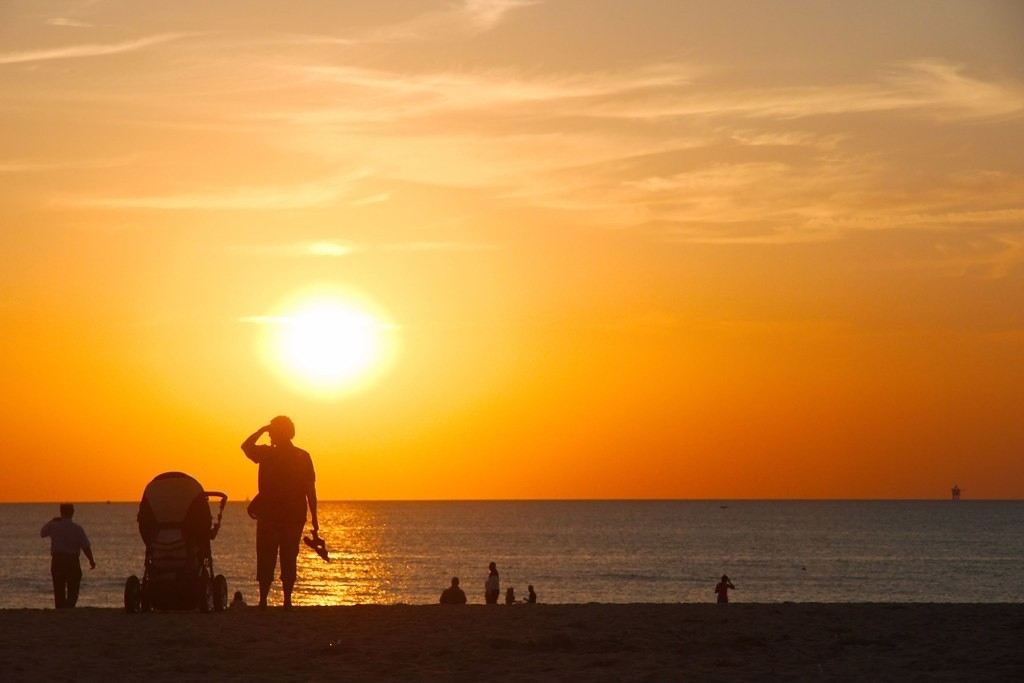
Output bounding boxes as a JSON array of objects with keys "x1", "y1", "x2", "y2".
[{"x1": 247, "y1": 491, "x2": 277, "y2": 519}]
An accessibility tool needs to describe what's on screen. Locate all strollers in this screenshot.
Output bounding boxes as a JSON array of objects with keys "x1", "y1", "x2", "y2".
[{"x1": 123, "y1": 468, "x2": 230, "y2": 618}]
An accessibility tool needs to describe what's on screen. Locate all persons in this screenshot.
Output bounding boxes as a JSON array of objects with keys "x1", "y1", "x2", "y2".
[
  {"x1": 715, "y1": 575, "x2": 735, "y2": 603},
  {"x1": 484, "y1": 562, "x2": 500, "y2": 605},
  {"x1": 40, "y1": 503, "x2": 96, "y2": 609},
  {"x1": 439, "y1": 576, "x2": 467, "y2": 604},
  {"x1": 505, "y1": 585, "x2": 536, "y2": 603},
  {"x1": 241, "y1": 415, "x2": 319, "y2": 606}
]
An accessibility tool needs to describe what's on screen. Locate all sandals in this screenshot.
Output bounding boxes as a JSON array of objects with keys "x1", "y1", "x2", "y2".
[{"x1": 304, "y1": 530, "x2": 329, "y2": 563}]
